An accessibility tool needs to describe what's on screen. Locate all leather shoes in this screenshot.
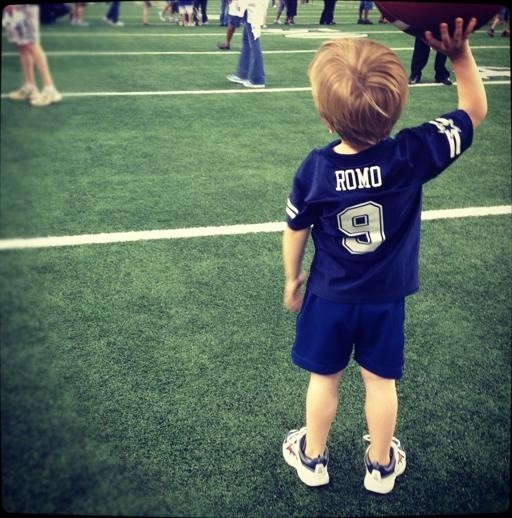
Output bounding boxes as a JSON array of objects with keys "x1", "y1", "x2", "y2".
[
  {"x1": 436, "y1": 76, "x2": 452, "y2": 85},
  {"x1": 407, "y1": 73, "x2": 422, "y2": 84}
]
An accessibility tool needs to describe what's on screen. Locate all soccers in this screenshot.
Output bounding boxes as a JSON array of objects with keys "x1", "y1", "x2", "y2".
[{"x1": 374, "y1": 0, "x2": 504, "y2": 40}]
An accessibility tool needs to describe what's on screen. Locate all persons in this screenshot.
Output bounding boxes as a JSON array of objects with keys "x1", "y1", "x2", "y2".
[
  {"x1": 407, "y1": 37, "x2": 451, "y2": 85},
  {"x1": 224, "y1": 1, "x2": 268, "y2": 88},
  {"x1": 1, "y1": 4, "x2": 63, "y2": 107},
  {"x1": 283, "y1": 18, "x2": 488, "y2": 494},
  {"x1": 218, "y1": 1, "x2": 252, "y2": 50}
]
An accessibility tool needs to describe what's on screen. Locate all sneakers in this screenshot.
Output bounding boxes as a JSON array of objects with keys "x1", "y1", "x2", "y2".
[
  {"x1": 7, "y1": 82, "x2": 39, "y2": 100},
  {"x1": 70, "y1": 11, "x2": 227, "y2": 27},
  {"x1": 242, "y1": 79, "x2": 265, "y2": 88},
  {"x1": 217, "y1": 43, "x2": 231, "y2": 49},
  {"x1": 29, "y1": 85, "x2": 62, "y2": 107},
  {"x1": 362, "y1": 436, "x2": 407, "y2": 495},
  {"x1": 274, "y1": 18, "x2": 390, "y2": 25},
  {"x1": 487, "y1": 30, "x2": 494, "y2": 37},
  {"x1": 282, "y1": 425, "x2": 331, "y2": 488},
  {"x1": 501, "y1": 31, "x2": 510, "y2": 37},
  {"x1": 226, "y1": 74, "x2": 248, "y2": 84}
]
